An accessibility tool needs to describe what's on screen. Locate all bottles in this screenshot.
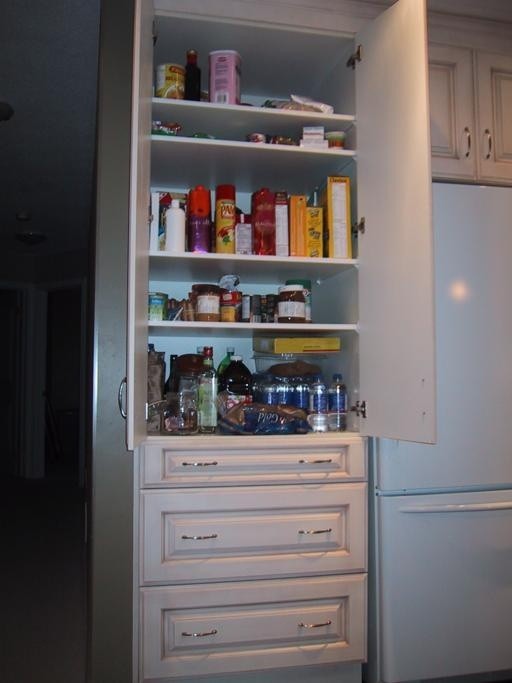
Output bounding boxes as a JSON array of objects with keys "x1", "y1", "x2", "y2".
[
  {"x1": 160, "y1": 391, "x2": 200, "y2": 434},
  {"x1": 192, "y1": 284, "x2": 220, "y2": 321},
  {"x1": 146, "y1": 343, "x2": 165, "y2": 404},
  {"x1": 165, "y1": 199, "x2": 186, "y2": 251},
  {"x1": 165, "y1": 354, "x2": 180, "y2": 392},
  {"x1": 253, "y1": 371, "x2": 346, "y2": 431},
  {"x1": 185, "y1": 49, "x2": 201, "y2": 100},
  {"x1": 196, "y1": 346, "x2": 251, "y2": 431}
]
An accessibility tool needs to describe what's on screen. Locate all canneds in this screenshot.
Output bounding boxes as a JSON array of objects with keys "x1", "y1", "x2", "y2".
[
  {"x1": 147, "y1": 291, "x2": 169, "y2": 321},
  {"x1": 190, "y1": 284, "x2": 221, "y2": 322},
  {"x1": 220, "y1": 290, "x2": 243, "y2": 306},
  {"x1": 220, "y1": 304, "x2": 241, "y2": 322},
  {"x1": 284, "y1": 279, "x2": 313, "y2": 324}
]
